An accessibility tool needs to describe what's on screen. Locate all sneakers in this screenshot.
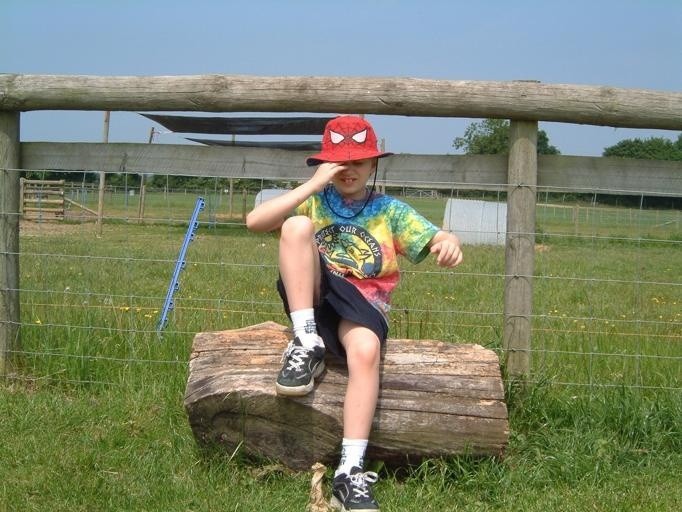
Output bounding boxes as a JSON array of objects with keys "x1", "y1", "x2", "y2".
[
  {"x1": 330, "y1": 467, "x2": 381, "y2": 511},
  {"x1": 276, "y1": 334, "x2": 325, "y2": 395}
]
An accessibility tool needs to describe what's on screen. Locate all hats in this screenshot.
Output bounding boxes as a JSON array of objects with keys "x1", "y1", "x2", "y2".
[{"x1": 306, "y1": 116, "x2": 393, "y2": 167}]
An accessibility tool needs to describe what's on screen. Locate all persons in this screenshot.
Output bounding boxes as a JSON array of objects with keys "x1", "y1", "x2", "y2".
[{"x1": 246, "y1": 116, "x2": 462, "y2": 512}]
